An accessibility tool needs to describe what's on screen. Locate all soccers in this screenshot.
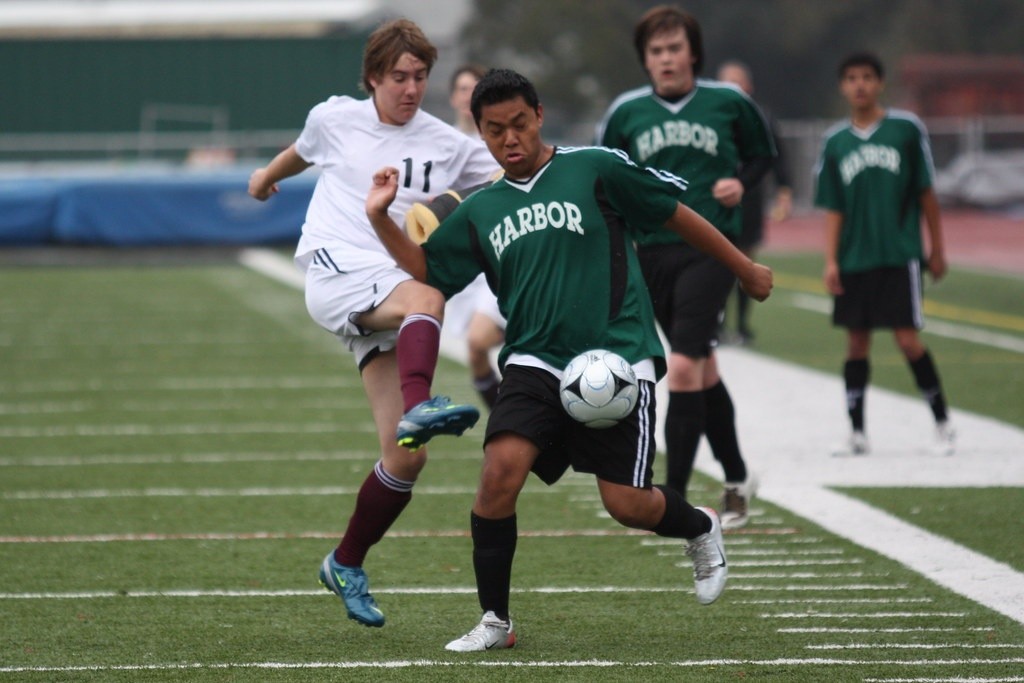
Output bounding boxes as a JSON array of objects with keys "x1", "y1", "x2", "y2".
[{"x1": 559, "y1": 348, "x2": 640, "y2": 432}]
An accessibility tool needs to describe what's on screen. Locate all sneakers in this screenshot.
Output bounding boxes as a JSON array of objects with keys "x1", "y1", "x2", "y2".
[
  {"x1": 318, "y1": 550, "x2": 385, "y2": 627},
  {"x1": 445, "y1": 610, "x2": 517, "y2": 652},
  {"x1": 683, "y1": 506, "x2": 729, "y2": 605},
  {"x1": 395, "y1": 396, "x2": 479, "y2": 453},
  {"x1": 720, "y1": 468, "x2": 759, "y2": 530}
]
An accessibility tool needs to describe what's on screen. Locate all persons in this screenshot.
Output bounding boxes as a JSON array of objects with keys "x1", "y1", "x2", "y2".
[
  {"x1": 592, "y1": 5, "x2": 780, "y2": 535},
  {"x1": 367, "y1": 66, "x2": 773, "y2": 653},
  {"x1": 246, "y1": 17, "x2": 505, "y2": 626},
  {"x1": 811, "y1": 44, "x2": 960, "y2": 459},
  {"x1": 709, "y1": 52, "x2": 797, "y2": 349}
]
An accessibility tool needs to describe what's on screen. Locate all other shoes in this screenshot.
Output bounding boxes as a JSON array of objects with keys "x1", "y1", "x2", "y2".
[
  {"x1": 734, "y1": 324, "x2": 754, "y2": 339},
  {"x1": 933, "y1": 420, "x2": 957, "y2": 454},
  {"x1": 830, "y1": 432, "x2": 870, "y2": 457}
]
